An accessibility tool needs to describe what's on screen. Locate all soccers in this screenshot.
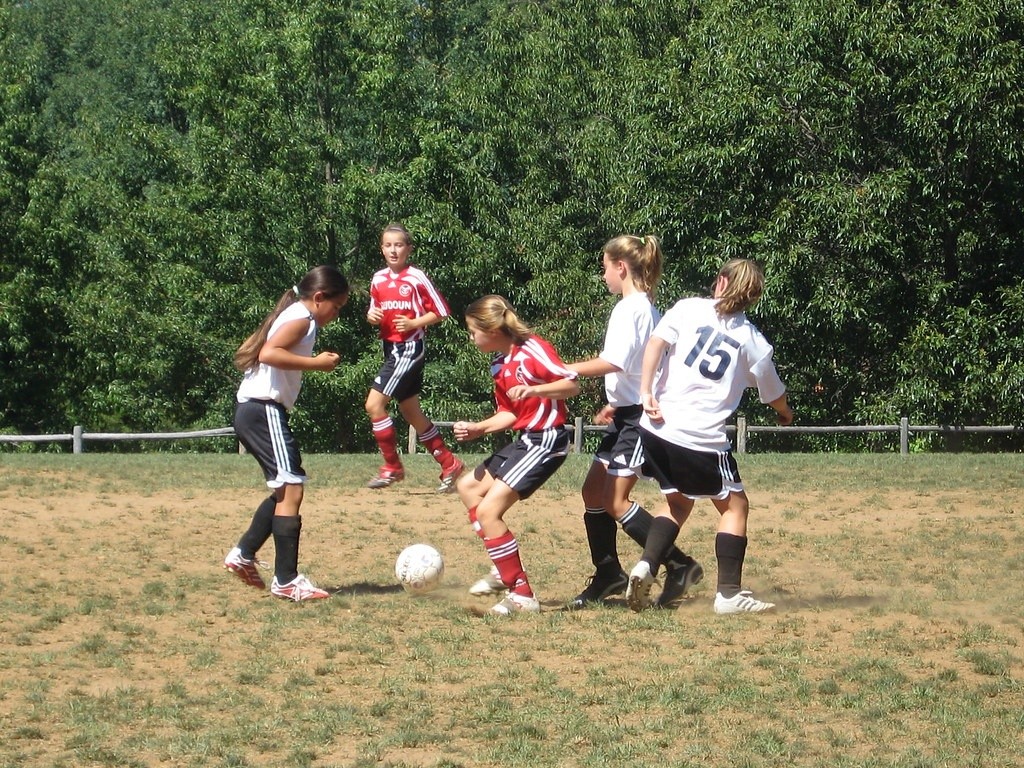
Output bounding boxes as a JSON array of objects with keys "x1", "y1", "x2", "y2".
[{"x1": 394, "y1": 542, "x2": 444, "y2": 592}]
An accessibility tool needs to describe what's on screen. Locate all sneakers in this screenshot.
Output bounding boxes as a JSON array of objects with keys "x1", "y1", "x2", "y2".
[
  {"x1": 626, "y1": 561, "x2": 654, "y2": 613},
  {"x1": 468, "y1": 566, "x2": 509, "y2": 595},
  {"x1": 657, "y1": 556, "x2": 704, "y2": 611},
  {"x1": 271, "y1": 573, "x2": 329, "y2": 601},
  {"x1": 435, "y1": 456, "x2": 466, "y2": 494},
  {"x1": 569, "y1": 568, "x2": 629, "y2": 607},
  {"x1": 368, "y1": 470, "x2": 404, "y2": 488},
  {"x1": 223, "y1": 547, "x2": 265, "y2": 589},
  {"x1": 490, "y1": 592, "x2": 540, "y2": 617},
  {"x1": 715, "y1": 590, "x2": 775, "y2": 615}
]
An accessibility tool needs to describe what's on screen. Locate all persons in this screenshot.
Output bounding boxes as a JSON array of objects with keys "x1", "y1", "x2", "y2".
[
  {"x1": 227, "y1": 265, "x2": 351, "y2": 605},
  {"x1": 364, "y1": 221, "x2": 469, "y2": 493},
  {"x1": 563, "y1": 234, "x2": 705, "y2": 614},
  {"x1": 625, "y1": 257, "x2": 793, "y2": 616},
  {"x1": 452, "y1": 294, "x2": 581, "y2": 617}
]
